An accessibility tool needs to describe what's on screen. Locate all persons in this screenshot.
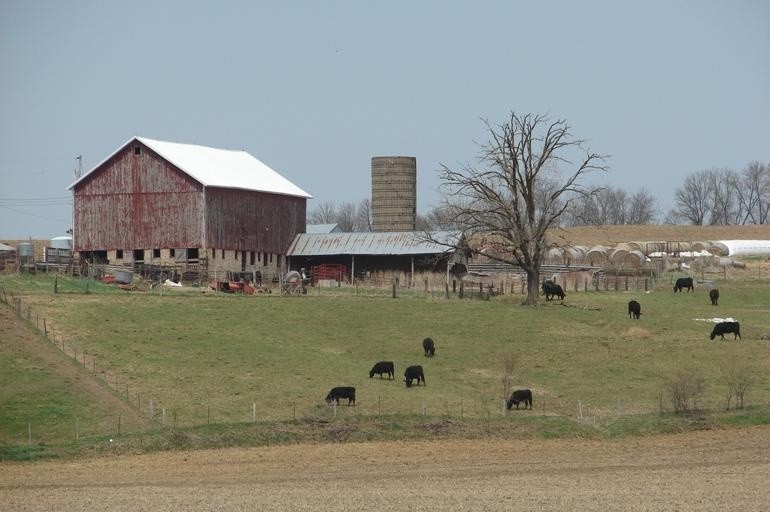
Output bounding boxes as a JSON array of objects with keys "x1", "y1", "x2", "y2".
[{"x1": 300, "y1": 267, "x2": 311, "y2": 294}]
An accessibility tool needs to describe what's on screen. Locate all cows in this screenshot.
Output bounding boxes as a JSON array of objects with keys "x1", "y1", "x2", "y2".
[
  {"x1": 709, "y1": 289, "x2": 719, "y2": 306},
  {"x1": 541, "y1": 279, "x2": 566, "y2": 302},
  {"x1": 325, "y1": 387, "x2": 356, "y2": 407},
  {"x1": 402, "y1": 365, "x2": 425, "y2": 387},
  {"x1": 369, "y1": 361, "x2": 394, "y2": 381},
  {"x1": 506, "y1": 389, "x2": 533, "y2": 410},
  {"x1": 628, "y1": 300, "x2": 641, "y2": 319},
  {"x1": 710, "y1": 321, "x2": 742, "y2": 341},
  {"x1": 673, "y1": 278, "x2": 694, "y2": 293},
  {"x1": 422, "y1": 337, "x2": 435, "y2": 358}
]
